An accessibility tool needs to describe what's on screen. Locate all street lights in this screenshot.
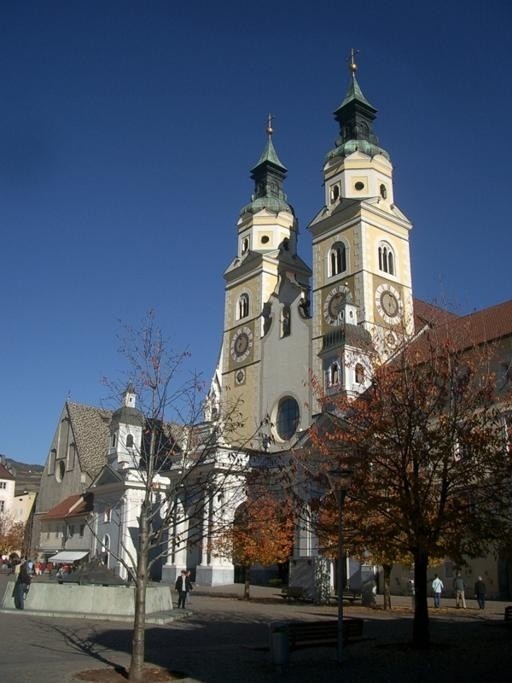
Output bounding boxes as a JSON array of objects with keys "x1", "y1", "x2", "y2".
[{"x1": 324, "y1": 465, "x2": 354, "y2": 658}]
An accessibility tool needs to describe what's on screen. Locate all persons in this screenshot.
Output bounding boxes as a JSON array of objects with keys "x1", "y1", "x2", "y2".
[
  {"x1": 1, "y1": 557, "x2": 86, "y2": 580},
  {"x1": 174, "y1": 569, "x2": 190, "y2": 609},
  {"x1": 127, "y1": 566, "x2": 133, "y2": 582},
  {"x1": 11, "y1": 565, "x2": 32, "y2": 612},
  {"x1": 186, "y1": 570, "x2": 193, "y2": 605},
  {"x1": 474, "y1": 575, "x2": 487, "y2": 611},
  {"x1": 432, "y1": 572, "x2": 444, "y2": 608},
  {"x1": 452, "y1": 570, "x2": 469, "y2": 609}
]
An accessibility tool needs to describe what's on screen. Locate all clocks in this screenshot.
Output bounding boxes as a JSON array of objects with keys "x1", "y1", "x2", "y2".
[
  {"x1": 322, "y1": 283, "x2": 354, "y2": 327},
  {"x1": 230, "y1": 325, "x2": 253, "y2": 363},
  {"x1": 374, "y1": 281, "x2": 405, "y2": 326}
]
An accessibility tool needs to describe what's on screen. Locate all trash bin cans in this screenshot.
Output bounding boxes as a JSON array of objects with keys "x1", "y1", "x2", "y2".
[
  {"x1": 362, "y1": 580, "x2": 377, "y2": 609},
  {"x1": 288, "y1": 556, "x2": 316, "y2": 601}
]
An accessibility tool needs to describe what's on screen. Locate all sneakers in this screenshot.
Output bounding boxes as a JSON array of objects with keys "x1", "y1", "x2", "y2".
[
  {"x1": 436, "y1": 606, "x2": 440, "y2": 608},
  {"x1": 182, "y1": 607, "x2": 186, "y2": 609},
  {"x1": 178, "y1": 606, "x2": 180, "y2": 609},
  {"x1": 479, "y1": 607, "x2": 484, "y2": 610},
  {"x1": 456, "y1": 606, "x2": 466, "y2": 610}
]
